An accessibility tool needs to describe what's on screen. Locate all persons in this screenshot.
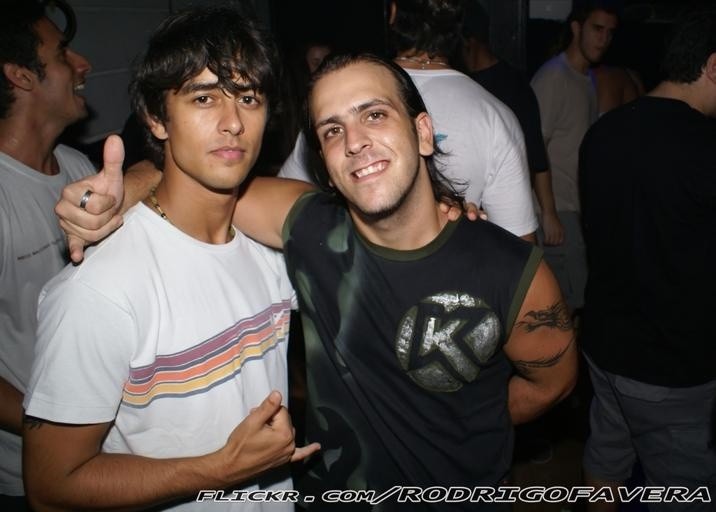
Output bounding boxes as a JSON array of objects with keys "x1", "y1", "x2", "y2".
[{"x1": 0, "y1": 0, "x2": 716, "y2": 512}]
